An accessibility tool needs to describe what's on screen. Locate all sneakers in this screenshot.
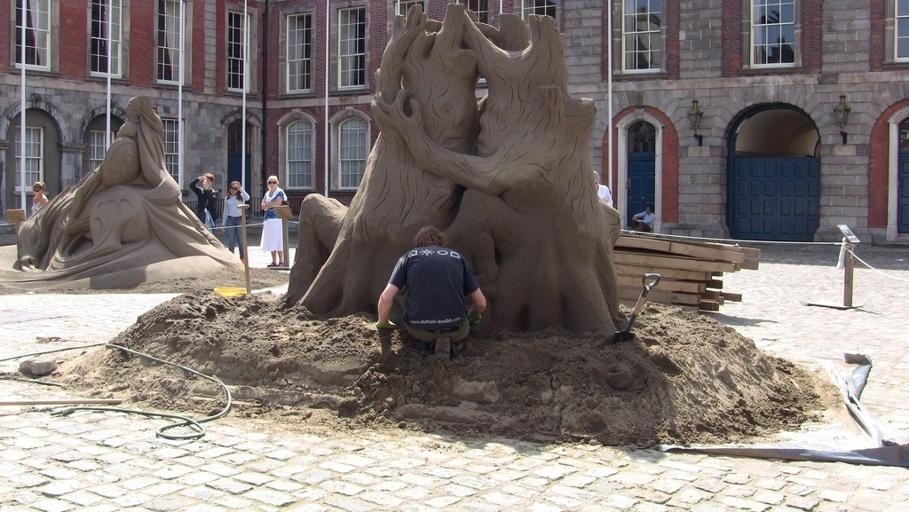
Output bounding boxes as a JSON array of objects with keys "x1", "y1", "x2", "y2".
[
  {"x1": 268, "y1": 262, "x2": 284, "y2": 267},
  {"x1": 431, "y1": 335, "x2": 453, "y2": 359}
]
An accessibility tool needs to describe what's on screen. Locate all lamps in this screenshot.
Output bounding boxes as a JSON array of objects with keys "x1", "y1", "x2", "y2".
[
  {"x1": 684, "y1": 96, "x2": 708, "y2": 148},
  {"x1": 831, "y1": 93, "x2": 854, "y2": 145}
]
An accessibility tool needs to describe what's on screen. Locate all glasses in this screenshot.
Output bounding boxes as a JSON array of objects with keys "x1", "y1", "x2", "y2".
[
  {"x1": 268, "y1": 181, "x2": 277, "y2": 184},
  {"x1": 230, "y1": 186, "x2": 237, "y2": 190}
]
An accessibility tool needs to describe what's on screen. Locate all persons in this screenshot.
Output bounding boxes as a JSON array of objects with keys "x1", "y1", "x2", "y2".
[
  {"x1": 30, "y1": 181, "x2": 49, "y2": 218},
  {"x1": 220, "y1": 180, "x2": 251, "y2": 265},
  {"x1": 375, "y1": 224, "x2": 488, "y2": 359},
  {"x1": 630, "y1": 205, "x2": 655, "y2": 233},
  {"x1": 259, "y1": 175, "x2": 289, "y2": 267},
  {"x1": 188, "y1": 172, "x2": 219, "y2": 240},
  {"x1": 592, "y1": 170, "x2": 615, "y2": 208}
]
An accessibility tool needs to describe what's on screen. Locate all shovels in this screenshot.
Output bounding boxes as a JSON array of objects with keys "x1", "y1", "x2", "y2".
[{"x1": 613, "y1": 273, "x2": 662, "y2": 345}]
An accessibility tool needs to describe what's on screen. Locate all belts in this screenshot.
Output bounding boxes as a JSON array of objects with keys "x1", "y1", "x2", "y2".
[{"x1": 428, "y1": 326, "x2": 459, "y2": 334}]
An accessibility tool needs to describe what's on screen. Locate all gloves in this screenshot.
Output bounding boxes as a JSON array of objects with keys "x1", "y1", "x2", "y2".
[
  {"x1": 375, "y1": 319, "x2": 397, "y2": 329},
  {"x1": 468, "y1": 311, "x2": 482, "y2": 324}
]
